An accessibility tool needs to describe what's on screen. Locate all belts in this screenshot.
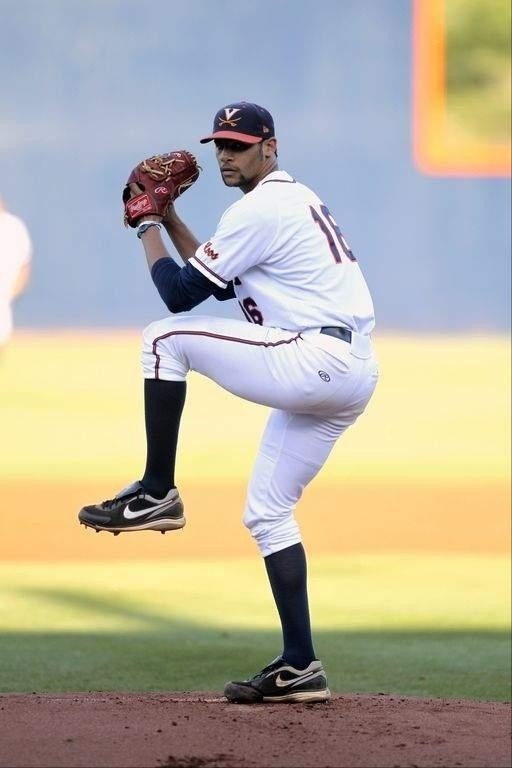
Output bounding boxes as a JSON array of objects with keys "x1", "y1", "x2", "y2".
[{"x1": 321, "y1": 327, "x2": 353, "y2": 343}]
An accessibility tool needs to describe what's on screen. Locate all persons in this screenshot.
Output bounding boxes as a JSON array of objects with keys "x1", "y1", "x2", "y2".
[{"x1": 78, "y1": 102, "x2": 379, "y2": 704}]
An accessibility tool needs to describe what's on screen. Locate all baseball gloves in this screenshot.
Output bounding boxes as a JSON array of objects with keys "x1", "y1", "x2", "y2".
[{"x1": 123, "y1": 151, "x2": 203, "y2": 228}]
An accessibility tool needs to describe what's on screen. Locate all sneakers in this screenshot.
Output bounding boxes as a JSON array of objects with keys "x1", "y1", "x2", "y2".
[
  {"x1": 78, "y1": 482, "x2": 186, "y2": 536},
  {"x1": 225, "y1": 657, "x2": 330, "y2": 703}
]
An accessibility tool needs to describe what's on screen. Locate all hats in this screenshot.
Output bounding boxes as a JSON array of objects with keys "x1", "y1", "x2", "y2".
[{"x1": 200, "y1": 101, "x2": 275, "y2": 144}]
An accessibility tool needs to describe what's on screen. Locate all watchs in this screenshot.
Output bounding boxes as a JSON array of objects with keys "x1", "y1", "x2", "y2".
[{"x1": 138, "y1": 220, "x2": 161, "y2": 239}]
